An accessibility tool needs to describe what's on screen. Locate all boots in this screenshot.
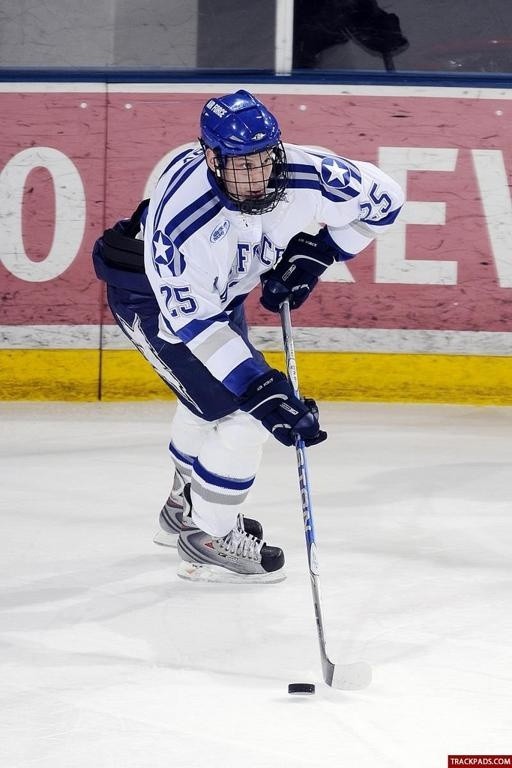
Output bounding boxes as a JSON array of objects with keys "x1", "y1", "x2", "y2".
[
  {"x1": 174, "y1": 480, "x2": 287, "y2": 577},
  {"x1": 159, "y1": 468, "x2": 267, "y2": 546}
]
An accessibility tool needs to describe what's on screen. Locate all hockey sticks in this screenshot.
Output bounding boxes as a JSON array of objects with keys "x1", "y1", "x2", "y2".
[{"x1": 279, "y1": 301, "x2": 373, "y2": 687}]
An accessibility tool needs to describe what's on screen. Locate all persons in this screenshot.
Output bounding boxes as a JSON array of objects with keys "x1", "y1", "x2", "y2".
[
  {"x1": 92, "y1": 90, "x2": 406, "y2": 574},
  {"x1": 197, "y1": 0, "x2": 408, "y2": 70}
]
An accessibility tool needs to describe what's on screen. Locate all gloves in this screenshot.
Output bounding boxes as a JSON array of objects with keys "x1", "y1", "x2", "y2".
[
  {"x1": 258, "y1": 231, "x2": 333, "y2": 314},
  {"x1": 237, "y1": 363, "x2": 331, "y2": 449}
]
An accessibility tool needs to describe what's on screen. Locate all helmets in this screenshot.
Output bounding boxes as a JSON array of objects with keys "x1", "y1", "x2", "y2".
[{"x1": 199, "y1": 89, "x2": 282, "y2": 157}]
